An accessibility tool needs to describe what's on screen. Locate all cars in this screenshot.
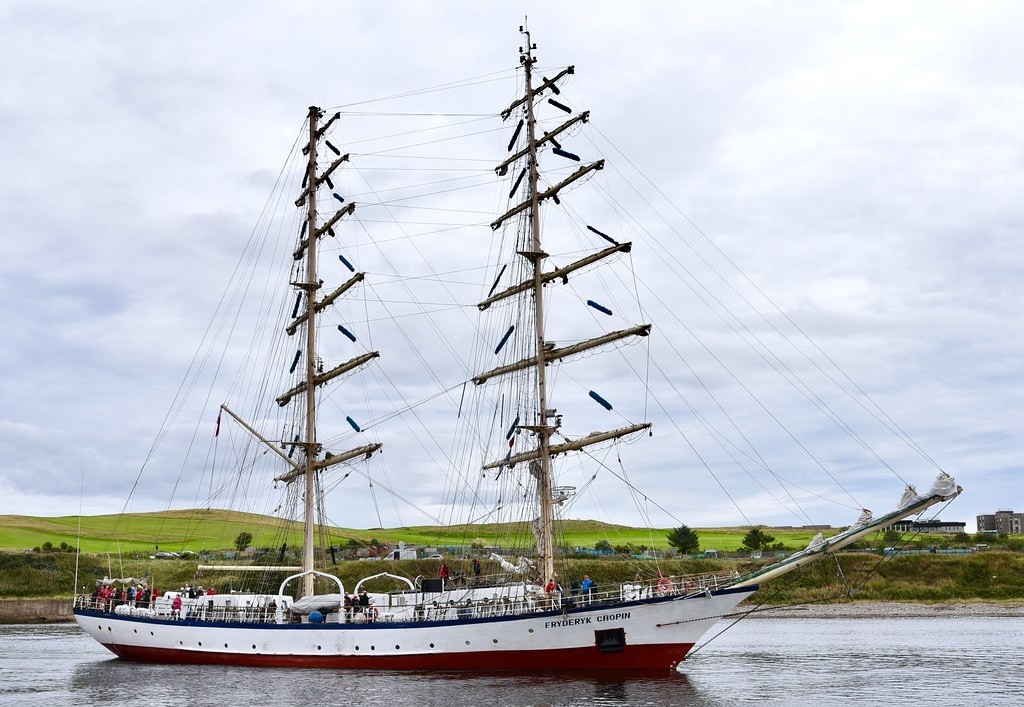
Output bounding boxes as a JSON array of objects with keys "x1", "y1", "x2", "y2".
[
  {"x1": 154, "y1": 551, "x2": 200, "y2": 561},
  {"x1": 421, "y1": 555, "x2": 444, "y2": 560}
]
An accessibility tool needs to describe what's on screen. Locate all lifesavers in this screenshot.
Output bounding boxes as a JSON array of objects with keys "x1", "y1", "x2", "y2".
[
  {"x1": 369, "y1": 607, "x2": 379, "y2": 619},
  {"x1": 658, "y1": 578, "x2": 673, "y2": 592}
]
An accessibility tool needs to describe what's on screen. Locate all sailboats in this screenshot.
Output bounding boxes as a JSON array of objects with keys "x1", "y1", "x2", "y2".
[{"x1": 74, "y1": 15, "x2": 965, "y2": 673}]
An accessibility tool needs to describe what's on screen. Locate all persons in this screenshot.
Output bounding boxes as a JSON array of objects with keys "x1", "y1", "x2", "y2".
[
  {"x1": 343, "y1": 591, "x2": 352, "y2": 624},
  {"x1": 180, "y1": 587, "x2": 187, "y2": 598},
  {"x1": 188, "y1": 585, "x2": 196, "y2": 598},
  {"x1": 173, "y1": 593, "x2": 182, "y2": 621},
  {"x1": 351, "y1": 595, "x2": 360, "y2": 617},
  {"x1": 90, "y1": 581, "x2": 160, "y2": 614},
  {"x1": 451, "y1": 570, "x2": 467, "y2": 590},
  {"x1": 632, "y1": 568, "x2": 644, "y2": 600},
  {"x1": 472, "y1": 558, "x2": 481, "y2": 587},
  {"x1": 438, "y1": 561, "x2": 451, "y2": 589},
  {"x1": 184, "y1": 583, "x2": 191, "y2": 592},
  {"x1": 359, "y1": 590, "x2": 373, "y2": 624},
  {"x1": 581, "y1": 574, "x2": 592, "y2": 607},
  {"x1": 555, "y1": 581, "x2": 564, "y2": 605},
  {"x1": 589, "y1": 580, "x2": 599, "y2": 604},
  {"x1": 570, "y1": 577, "x2": 580, "y2": 608},
  {"x1": 207, "y1": 586, "x2": 217, "y2": 595},
  {"x1": 268, "y1": 599, "x2": 277, "y2": 625},
  {"x1": 195, "y1": 585, "x2": 204, "y2": 599},
  {"x1": 545, "y1": 578, "x2": 555, "y2": 612}
]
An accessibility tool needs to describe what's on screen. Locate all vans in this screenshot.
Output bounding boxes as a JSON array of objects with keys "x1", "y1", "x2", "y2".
[{"x1": 383, "y1": 549, "x2": 418, "y2": 561}]
